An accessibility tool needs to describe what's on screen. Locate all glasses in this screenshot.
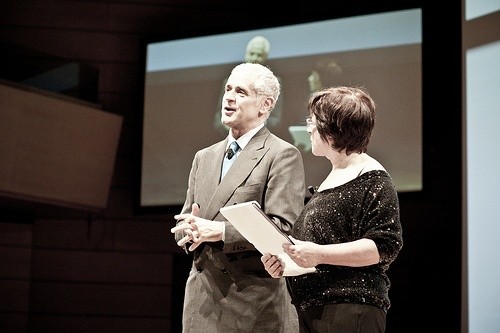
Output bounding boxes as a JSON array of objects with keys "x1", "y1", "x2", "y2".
[{"x1": 305, "y1": 117, "x2": 317, "y2": 128}]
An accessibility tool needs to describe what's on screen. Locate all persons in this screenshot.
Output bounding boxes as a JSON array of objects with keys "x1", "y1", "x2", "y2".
[
  {"x1": 288, "y1": 58, "x2": 342, "y2": 187},
  {"x1": 169, "y1": 60, "x2": 306, "y2": 333},
  {"x1": 259, "y1": 85, "x2": 403, "y2": 333},
  {"x1": 215, "y1": 35, "x2": 283, "y2": 130}
]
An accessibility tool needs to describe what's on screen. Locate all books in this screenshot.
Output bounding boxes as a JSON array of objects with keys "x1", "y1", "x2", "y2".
[
  {"x1": 288, "y1": 125, "x2": 315, "y2": 152},
  {"x1": 220, "y1": 200, "x2": 316, "y2": 278}
]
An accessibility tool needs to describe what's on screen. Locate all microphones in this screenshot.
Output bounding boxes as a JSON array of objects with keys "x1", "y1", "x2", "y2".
[
  {"x1": 225, "y1": 149, "x2": 235, "y2": 160},
  {"x1": 308, "y1": 185, "x2": 314, "y2": 195}
]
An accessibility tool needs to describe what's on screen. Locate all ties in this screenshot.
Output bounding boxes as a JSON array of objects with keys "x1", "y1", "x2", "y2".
[{"x1": 220, "y1": 142, "x2": 239, "y2": 184}]
